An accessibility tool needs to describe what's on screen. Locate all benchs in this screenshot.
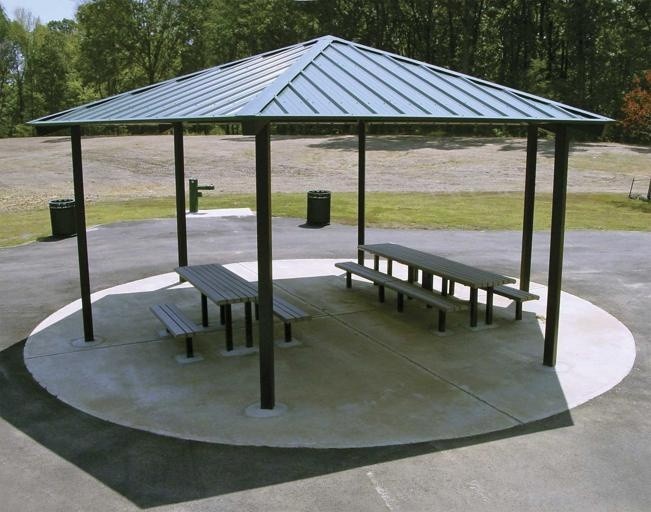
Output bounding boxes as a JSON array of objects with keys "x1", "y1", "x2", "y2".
[
  {"x1": 148, "y1": 295, "x2": 313, "y2": 360},
  {"x1": 334, "y1": 261, "x2": 542, "y2": 333}
]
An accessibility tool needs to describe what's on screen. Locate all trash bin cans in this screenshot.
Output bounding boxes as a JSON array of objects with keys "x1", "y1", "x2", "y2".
[
  {"x1": 48, "y1": 199, "x2": 77, "y2": 239},
  {"x1": 307, "y1": 190, "x2": 331, "y2": 226}
]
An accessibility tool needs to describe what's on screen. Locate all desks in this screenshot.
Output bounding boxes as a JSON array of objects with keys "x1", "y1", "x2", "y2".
[
  {"x1": 357, "y1": 242, "x2": 517, "y2": 328},
  {"x1": 173, "y1": 262, "x2": 260, "y2": 352}
]
